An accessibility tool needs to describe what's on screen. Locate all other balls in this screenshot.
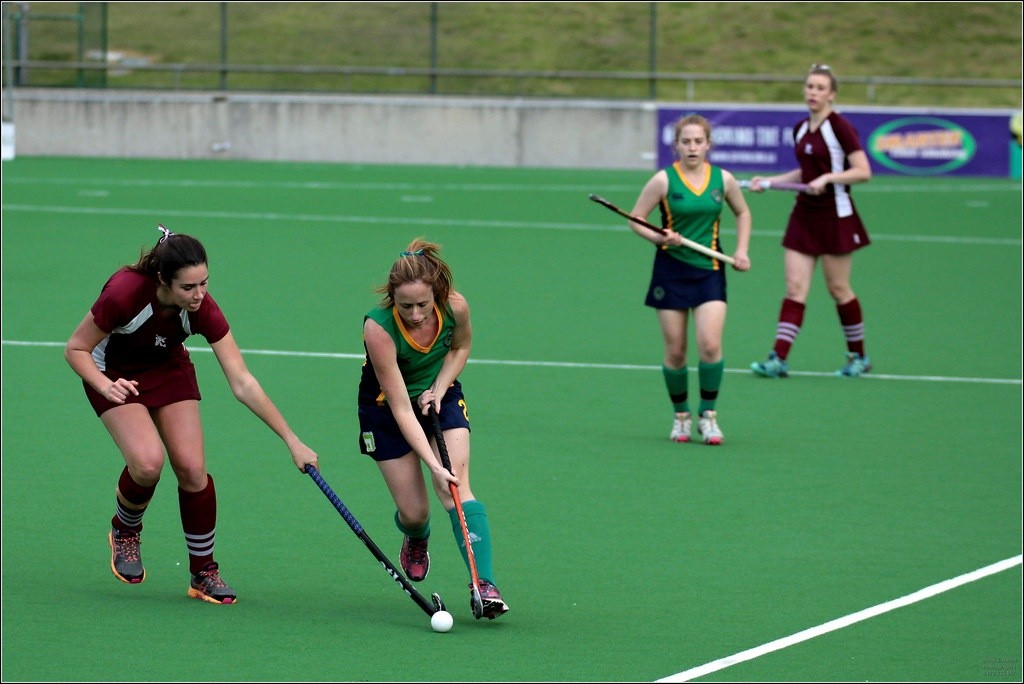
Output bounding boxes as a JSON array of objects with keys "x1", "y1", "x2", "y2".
[{"x1": 431, "y1": 610, "x2": 454, "y2": 632}]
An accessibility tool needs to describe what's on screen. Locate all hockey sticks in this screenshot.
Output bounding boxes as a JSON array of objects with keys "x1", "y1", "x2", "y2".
[
  {"x1": 588, "y1": 193, "x2": 741, "y2": 267},
  {"x1": 734, "y1": 178, "x2": 825, "y2": 194},
  {"x1": 427, "y1": 391, "x2": 484, "y2": 620},
  {"x1": 305, "y1": 463, "x2": 447, "y2": 619}
]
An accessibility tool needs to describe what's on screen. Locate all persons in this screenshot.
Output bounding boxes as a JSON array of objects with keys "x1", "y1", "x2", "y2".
[
  {"x1": 628, "y1": 114, "x2": 751, "y2": 446},
  {"x1": 357, "y1": 236, "x2": 511, "y2": 621},
  {"x1": 63, "y1": 234, "x2": 320, "y2": 605},
  {"x1": 749, "y1": 64, "x2": 872, "y2": 380}
]
